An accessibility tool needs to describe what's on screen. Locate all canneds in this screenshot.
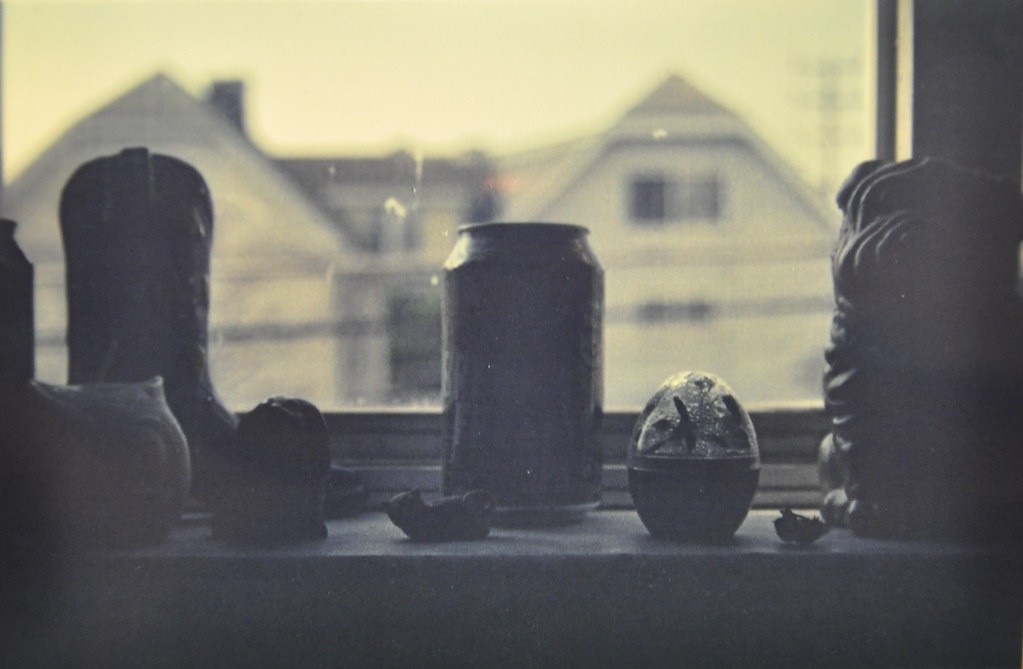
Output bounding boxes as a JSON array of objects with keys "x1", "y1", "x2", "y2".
[{"x1": 442, "y1": 221, "x2": 608, "y2": 521}]
